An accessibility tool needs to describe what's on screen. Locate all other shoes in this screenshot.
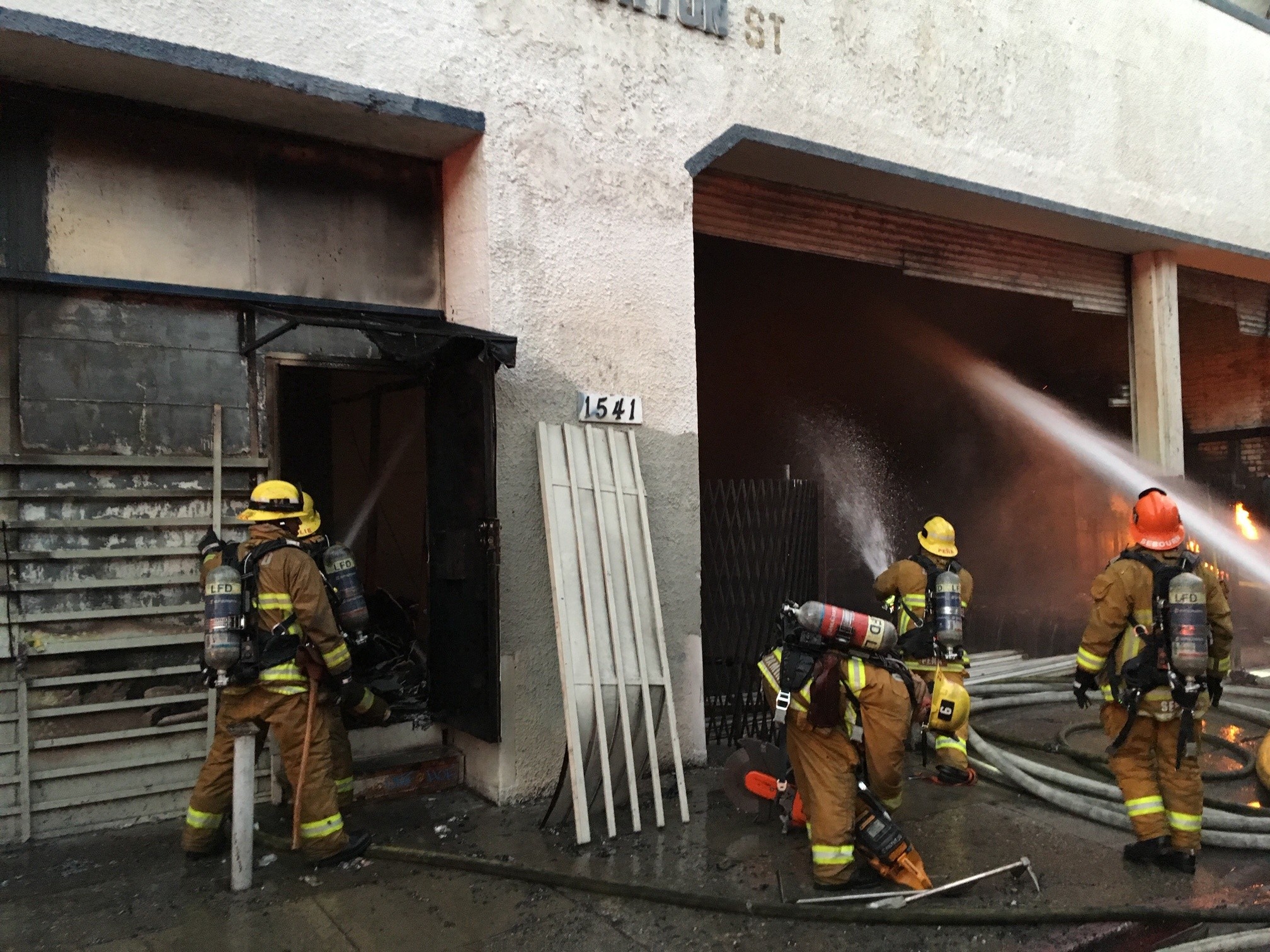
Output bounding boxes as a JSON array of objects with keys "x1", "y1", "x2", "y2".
[
  {"x1": 340, "y1": 807, "x2": 351, "y2": 816},
  {"x1": 1124, "y1": 835, "x2": 1171, "y2": 861},
  {"x1": 185, "y1": 835, "x2": 231, "y2": 860},
  {"x1": 1158, "y1": 849, "x2": 1198, "y2": 873},
  {"x1": 938, "y1": 764, "x2": 978, "y2": 784},
  {"x1": 813, "y1": 864, "x2": 881, "y2": 889},
  {"x1": 304, "y1": 832, "x2": 371, "y2": 868}
]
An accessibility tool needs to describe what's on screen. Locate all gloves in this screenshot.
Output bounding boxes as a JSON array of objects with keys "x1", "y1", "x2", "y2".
[
  {"x1": 197, "y1": 525, "x2": 221, "y2": 556},
  {"x1": 384, "y1": 712, "x2": 401, "y2": 725},
  {"x1": 1206, "y1": 674, "x2": 1222, "y2": 708},
  {"x1": 1072, "y1": 668, "x2": 1099, "y2": 708},
  {"x1": 335, "y1": 670, "x2": 356, "y2": 704}
]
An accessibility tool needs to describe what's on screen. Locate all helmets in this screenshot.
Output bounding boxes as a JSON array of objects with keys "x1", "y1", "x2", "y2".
[
  {"x1": 234, "y1": 479, "x2": 309, "y2": 520},
  {"x1": 1128, "y1": 488, "x2": 1185, "y2": 550},
  {"x1": 928, "y1": 659, "x2": 971, "y2": 731},
  {"x1": 296, "y1": 491, "x2": 320, "y2": 537},
  {"x1": 917, "y1": 515, "x2": 959, "y2": 557}
]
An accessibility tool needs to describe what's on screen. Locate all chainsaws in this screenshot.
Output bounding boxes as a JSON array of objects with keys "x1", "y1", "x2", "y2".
[{"x1": 725, "y1": 741, "x2": 796, "y2": 825}]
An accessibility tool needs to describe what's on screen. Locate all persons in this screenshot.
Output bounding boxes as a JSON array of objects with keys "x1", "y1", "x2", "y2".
[
  {"x1": 873, "y1": 516, "x2": 977, "y2": 780},
  {"x1": 1072, "y1": 489, "x2": 1233, "y2": 875},
  {"x1": 757, "y1": 643, "x2": 971, "y2": 892},
  {"x1": 180, "y1": 480, "x2": 391, "y2": 868}
]
